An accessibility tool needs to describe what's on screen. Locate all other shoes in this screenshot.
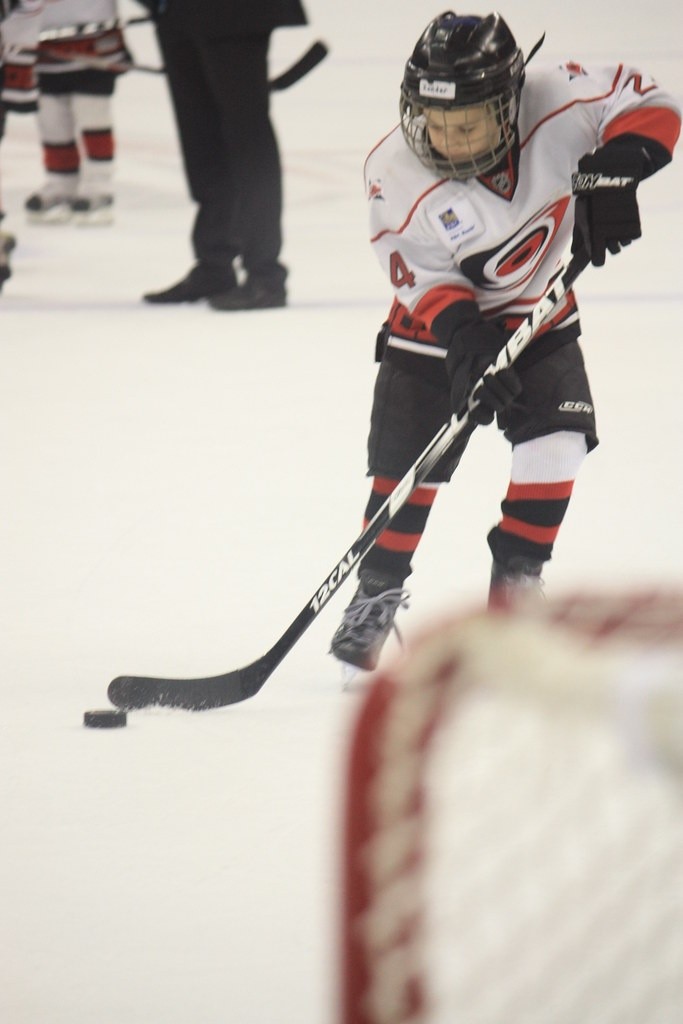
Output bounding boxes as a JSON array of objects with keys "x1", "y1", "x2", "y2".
[
  {"x1": 142, "y1": 256, "x2": 238, "y2": 304},
  {"x1": 208, "y1": 278, "x2": 285, "y2": 308}
]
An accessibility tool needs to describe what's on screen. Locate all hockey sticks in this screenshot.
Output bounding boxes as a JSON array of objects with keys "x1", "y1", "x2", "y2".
[
  {"x1": 16, "y1": 40, "x2": 327, "y2": 91},
  {"x1": 108, "y1": 248, "x2": 592, "y2": 710}
]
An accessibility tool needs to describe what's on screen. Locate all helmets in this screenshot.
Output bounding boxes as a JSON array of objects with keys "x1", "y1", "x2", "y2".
[{"x1": 397, "y1": 12, "x2": 528, "y2": 181}]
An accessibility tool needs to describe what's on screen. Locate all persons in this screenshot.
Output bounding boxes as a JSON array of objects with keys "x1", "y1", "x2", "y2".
[
  {"x1": 332, "y1": 11, "x2": 682, "y2": 690},
  {"x1": 0, "y1": 0, "x2": 133, "y2": 288},
  {"x1": 133, "y1": 0, "x2": 309, "y2": 310}
]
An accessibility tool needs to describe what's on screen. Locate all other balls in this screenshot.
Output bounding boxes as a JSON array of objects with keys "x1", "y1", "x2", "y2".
[{"x1": 83, "y1": 710, "x2": 127, "y2": 729}]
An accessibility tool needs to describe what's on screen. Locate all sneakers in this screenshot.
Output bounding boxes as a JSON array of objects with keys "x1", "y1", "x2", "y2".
[
  {"x1": 64, "y1": 182, "x2": 120, "y2": 227},
  {"x1": 25, "y1": 170, "x2": 80, "y2": 224},
  {"x1": 325, "y1": 573, "x2": 410, "y2": 694}
]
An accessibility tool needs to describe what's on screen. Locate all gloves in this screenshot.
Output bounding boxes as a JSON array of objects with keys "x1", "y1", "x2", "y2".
[
  {"x1": 431, "y1": 299, "x2": 524, "y2": 426},
  {"x1": 571, "y1": 150, "x2": 654, "y2": 266}
]
